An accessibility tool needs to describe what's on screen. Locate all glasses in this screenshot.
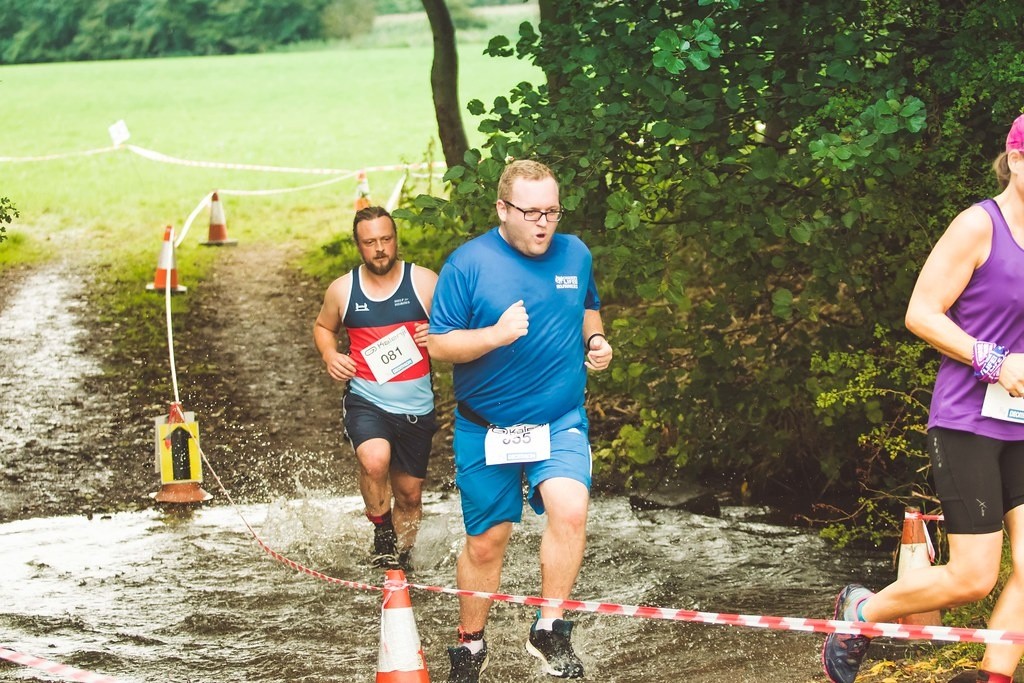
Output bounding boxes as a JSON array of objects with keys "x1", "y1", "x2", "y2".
[{"x1": 503, "y1": 199, "x2": 565, "y2": 222}]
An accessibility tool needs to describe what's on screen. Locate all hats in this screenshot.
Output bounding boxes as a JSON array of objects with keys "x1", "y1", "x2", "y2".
[{"x1": 1004, "y1": 114, "x2": 1024, "y2": 156}]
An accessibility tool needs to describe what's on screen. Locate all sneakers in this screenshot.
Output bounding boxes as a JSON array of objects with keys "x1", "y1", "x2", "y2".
[
  {"x1": 371, "y1": 525, "x2": 397, "y2": 567},
  {"x1": 524, "y1": 617, "x2": 589, "y2": 677},
  {"x1": 447, "y1": 641, "x2": 491, "y2": 683},
  {"x1": 393, "y1": 548, "x2": 417, "y2": 572},
  {"x1": 823, "y1": 585, "x2": 875, "y2": 681},
  {"x1": 946, "y1": 668, "x2": 987, "y2": 683}
]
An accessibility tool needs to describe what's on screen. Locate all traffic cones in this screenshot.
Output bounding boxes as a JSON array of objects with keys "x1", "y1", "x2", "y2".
[
  {"x1": 199, "y1": 189, "x2": 236, "y2": 244},
  {"x1": 375, "y1": 570, "x2": 431, "y2": 683},
  {"x1": 153, "y1": 401, "x2": 214, "y2": 503},
  {"x1": 895, "y1": 504, "x2": 944, "y2": 631},
  {"x1": 355, "y1": 169, "x2": 373, "y2": 214},
  {"x1": 145, "y1": 225, "x2": 187, "y2": 292}
]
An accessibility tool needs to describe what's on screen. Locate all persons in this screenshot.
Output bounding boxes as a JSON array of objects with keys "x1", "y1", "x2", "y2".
[
  {"x1": 427, "y1": 158, "x2": 614, "y2": 682},
  {"x1": 821, "y1": 111, "x2": 1024, "y2": 683},
  {"x1": 313, "y1": 206, "x2": 439, "y2": 566}
]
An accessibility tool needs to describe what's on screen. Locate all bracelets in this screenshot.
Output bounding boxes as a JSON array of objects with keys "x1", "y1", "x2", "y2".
[
  {"x1": 587, "y1": 334, "x2": 608, "y2": 351},
  {"x1": 973, "y1": 341, "x2": 1010, "y2": 384}
]
